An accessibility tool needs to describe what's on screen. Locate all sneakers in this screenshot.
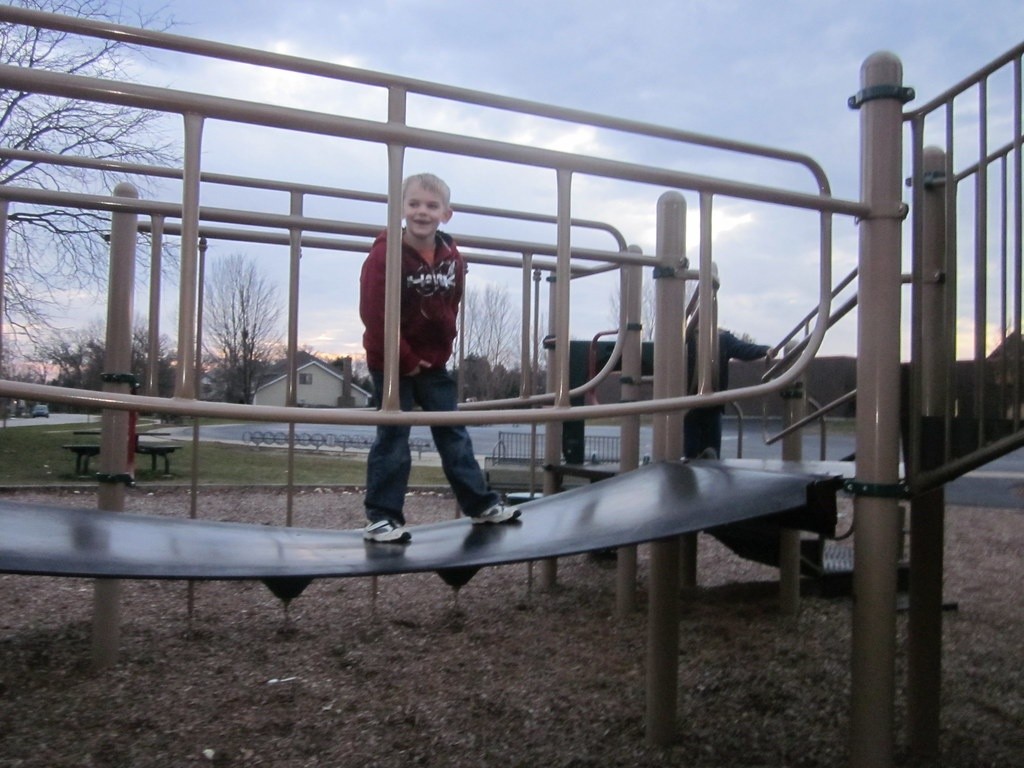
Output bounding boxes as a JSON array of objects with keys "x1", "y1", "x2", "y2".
[
  {"x1": 364, "y1": 518, "x2": 412, "y2": 543},
  {"x1": 472, "y1": 501, "x2": 522, "y2": 525}
]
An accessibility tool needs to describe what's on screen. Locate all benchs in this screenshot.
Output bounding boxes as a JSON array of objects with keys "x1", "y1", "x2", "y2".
[
  {"x1": 63, "y1": 444, "x2": 183, "y2": 478},
  {"x1": 485, "y1": 431, "x2": 621, "y2": 502}
]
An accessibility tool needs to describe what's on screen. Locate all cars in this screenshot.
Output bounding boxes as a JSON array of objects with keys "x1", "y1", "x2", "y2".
[{"x1": 32, "y1": 405, "x2": 49, "y2": 418}]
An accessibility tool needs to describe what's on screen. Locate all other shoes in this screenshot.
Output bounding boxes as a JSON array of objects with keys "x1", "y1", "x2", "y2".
[{"x1": 698, "y1": 447, "x2": 717, "y2": 461}]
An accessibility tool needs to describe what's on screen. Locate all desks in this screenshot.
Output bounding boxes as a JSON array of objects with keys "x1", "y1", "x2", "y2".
[{"x1": 73, "y1": 431, "x2": 170, "y2": 446}]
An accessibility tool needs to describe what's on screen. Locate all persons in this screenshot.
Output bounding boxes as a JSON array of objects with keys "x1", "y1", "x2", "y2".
[
  {"x1": 689, "y1": 312, "x2": 778, "y2": 460},
  {"x1": 358, "y1": 173, "x2": 521, "y2": 543}
]
